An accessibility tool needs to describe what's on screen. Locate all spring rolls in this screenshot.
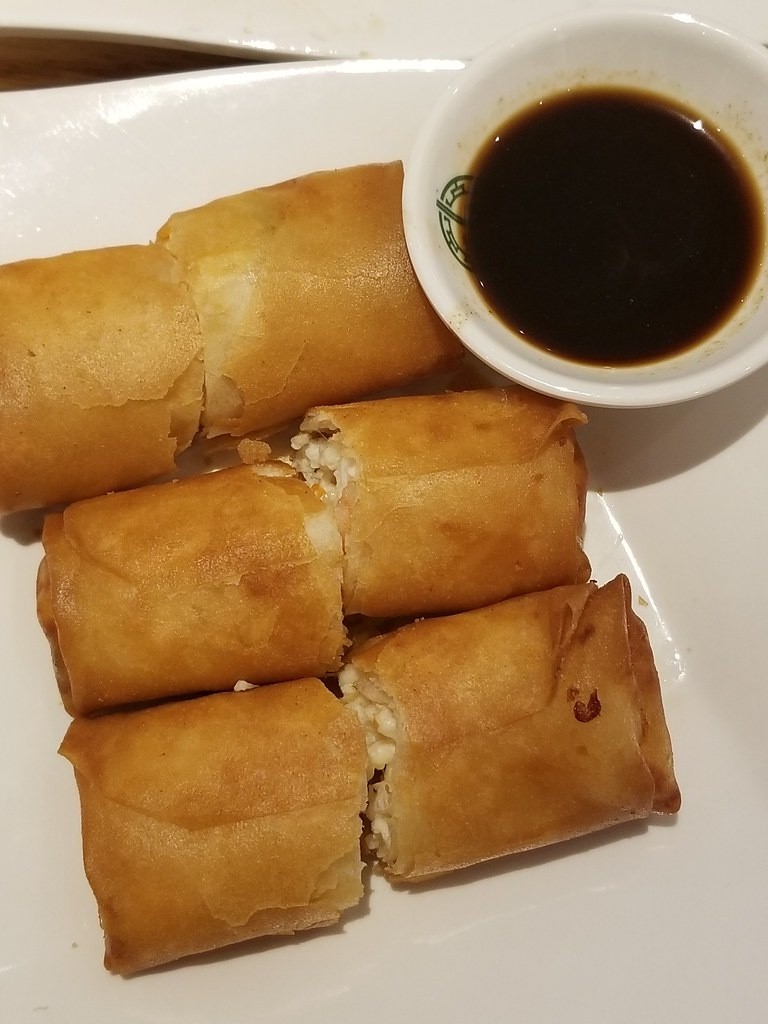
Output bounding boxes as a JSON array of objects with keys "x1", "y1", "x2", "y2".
[{"x1": 0, "y1": 158, "x2": 683, "y2": 975}]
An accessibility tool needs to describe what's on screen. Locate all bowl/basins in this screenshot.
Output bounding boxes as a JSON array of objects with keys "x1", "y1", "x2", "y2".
[{"x1": 400, "y1": 10, "x2": 768, "y2": 408}]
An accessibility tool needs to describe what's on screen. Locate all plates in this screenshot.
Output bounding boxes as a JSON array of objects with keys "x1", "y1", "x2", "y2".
[{"x1": 0, "y1": 59, "x2": 768, "y2": 1024}]
